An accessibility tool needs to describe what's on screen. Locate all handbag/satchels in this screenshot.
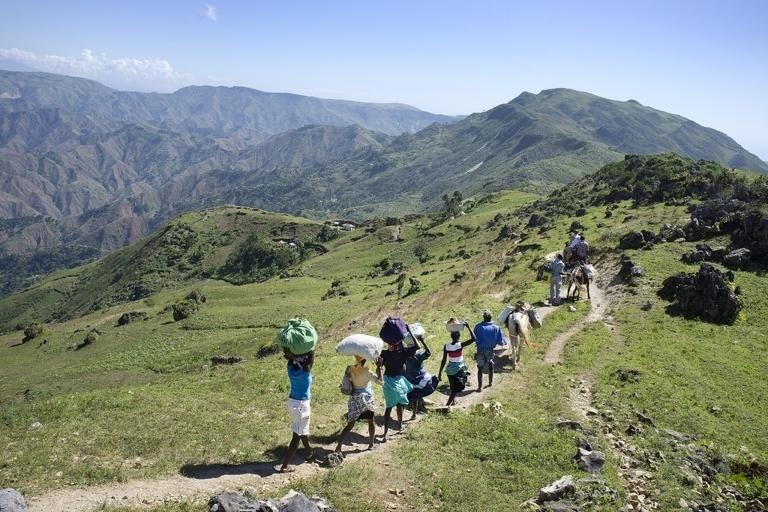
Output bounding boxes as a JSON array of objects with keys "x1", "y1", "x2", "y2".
[
  {"x1": 341, "y1": 366, "x2": 353, "y2": 395},
  {"x1": 497, "y1": 305, "x2": 516, "y2": 324},
  {"x1": 528, "y1": 305, "x2": 542, "y2": 329}
]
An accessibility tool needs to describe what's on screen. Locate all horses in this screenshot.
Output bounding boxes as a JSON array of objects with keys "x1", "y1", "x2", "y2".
[
  {"x1": 507, "y1": 302, "x2": 535, "y2": 370},
  {"x1": 566, "y1": 266, "x2": 590, "y2": 302}
]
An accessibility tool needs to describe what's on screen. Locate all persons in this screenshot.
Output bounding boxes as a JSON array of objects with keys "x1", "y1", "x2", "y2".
[
  {"x1": 550, "y1": 230, "x2": 589, "y2": 302},
  {"x1": 281, "y1": 346, "x2": 317, "y2": 472},
  {"x1": 473, "y1": 311, "x2": 507, "y2": 392},
  {"x1": 438, "y1": 321, "x2": 476, "y2": 406},
  {"x1": 335, "y1": 354, "x2": 382, "y2": 452},
  {"x1": 379, "y1": 324, "x2": 437, "y2": 442}
]
